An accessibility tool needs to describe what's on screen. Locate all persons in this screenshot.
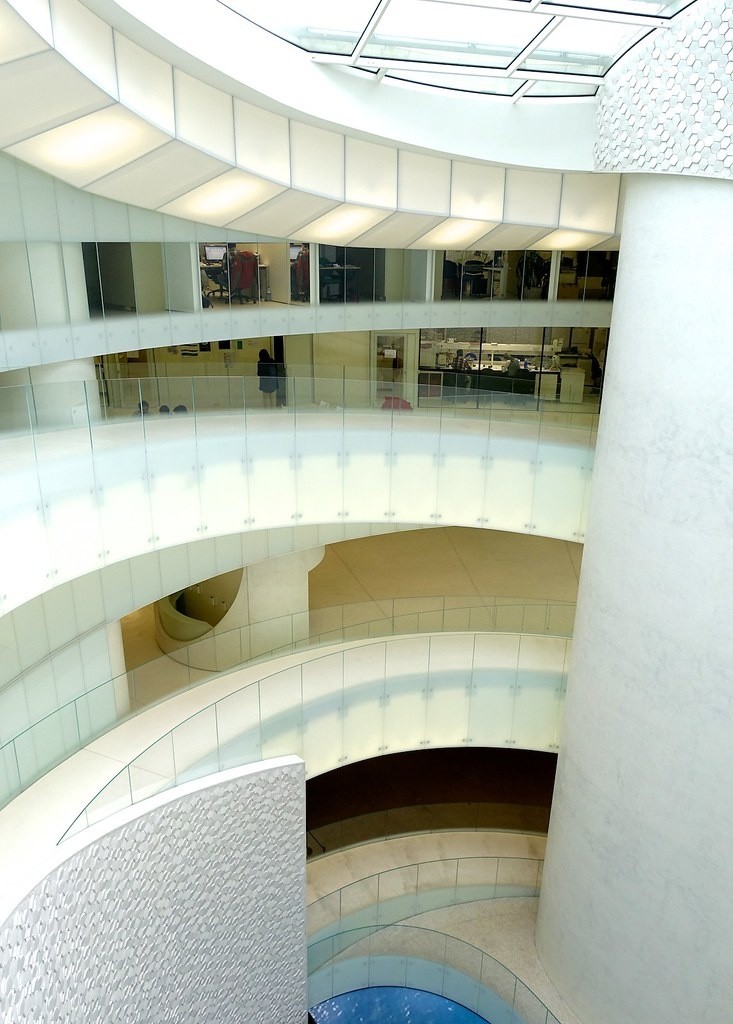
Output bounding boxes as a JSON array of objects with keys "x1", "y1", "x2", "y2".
[
  {"x1": 451, "y1": 348, "x2": 473, "y2": 391},
  {"x1": 172, "y1": 405, "x2": 189, "y2": 415},
  {"x1": 505, "y1": 352, "x2": 520, "y2": 378},
  {"x1": 159, "y1": 404, "x2": 170, "y2": 414},
  {"x1": 255, "y1": 348, "x2": 280, "y2": 409},
  {"x1": 131, "y1": 400, "x2": 153, "y2": 418},
  {"x1": 222, "y1": 243, "x2": 243, "y2": 273},
  {"x1": 296, "y1": 242, "x2": 310, "y2": 301}
]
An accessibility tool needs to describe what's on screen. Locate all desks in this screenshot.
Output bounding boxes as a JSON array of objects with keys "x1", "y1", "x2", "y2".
[
  {"x1": 198, "y1": 260, "x2": 269, "y2": 302},
  {"x1": 319, "y1": 261, "x2": 363, "y2": 303}
]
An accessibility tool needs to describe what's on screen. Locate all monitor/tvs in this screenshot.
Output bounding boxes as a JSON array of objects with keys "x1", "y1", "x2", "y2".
[
  {"x1": 435, "y1": 352, "x2": 449, "y2": 365},
  {"x1": 290, "y1": 245, "x2": 303, "y2": 261},
  {"x1": 203, "y1": 244, "x2": 227, "y2": 261}
]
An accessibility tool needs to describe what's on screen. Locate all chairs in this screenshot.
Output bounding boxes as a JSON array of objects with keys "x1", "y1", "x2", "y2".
[
  {"x1": 206, "y1": 269, "x2": 231, "y2": 300},
  {"x1": 226, "y1": 261, "x2": 259, "y2": 306}
]
